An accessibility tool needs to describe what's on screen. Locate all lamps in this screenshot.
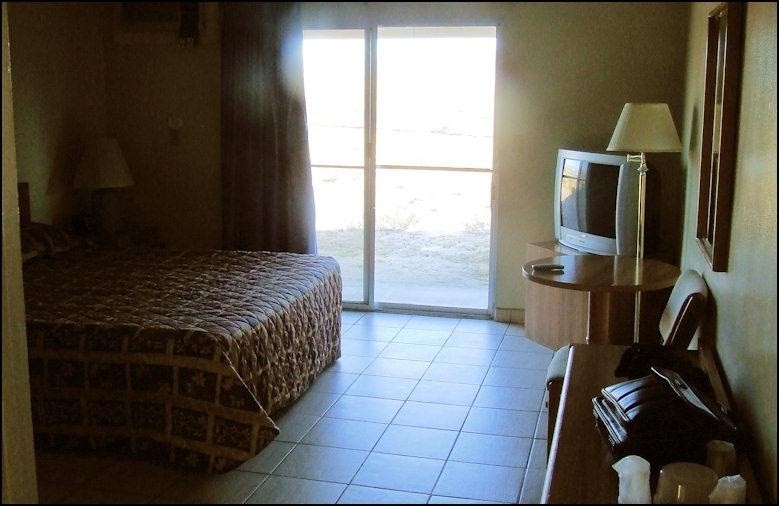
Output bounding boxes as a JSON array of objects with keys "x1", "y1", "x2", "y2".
[
  {"x1": 72, "y1": 132, "x2": 134, "y2": 229},
  {"x1": 605, "y1": 102, "x2": 681, "y2": 344}
]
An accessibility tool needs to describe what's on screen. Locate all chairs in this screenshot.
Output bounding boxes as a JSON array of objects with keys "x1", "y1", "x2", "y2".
[{"x1": 543, "y1": 270, "x2": 708, "y2": 465}]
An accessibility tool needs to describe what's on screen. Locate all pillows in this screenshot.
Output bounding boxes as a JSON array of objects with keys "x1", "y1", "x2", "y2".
[{"x1": 21, "y1": 219, "x2": 70, "y2": 263}]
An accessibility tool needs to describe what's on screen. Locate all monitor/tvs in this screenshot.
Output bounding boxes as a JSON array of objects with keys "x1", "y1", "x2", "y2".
[{"x1": 554, "y1": 149, "x2": 661, "y2": 257}]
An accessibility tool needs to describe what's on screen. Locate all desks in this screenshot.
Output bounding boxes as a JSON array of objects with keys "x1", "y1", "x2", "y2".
[
  {"x1": 524, "y1": 239, "x2": 609, "y2": 353},
  {"x1": 522, "y1": 255, "x2": 679, "y2": 292},
  {"x1": 540, "y1": 345, "x2": 762, "y2": 505}
]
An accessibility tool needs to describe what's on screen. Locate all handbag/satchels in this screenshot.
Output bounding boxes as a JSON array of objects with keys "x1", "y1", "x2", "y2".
[{"x1": 592, "y1": 366, "x2": 720, "y2": 459}]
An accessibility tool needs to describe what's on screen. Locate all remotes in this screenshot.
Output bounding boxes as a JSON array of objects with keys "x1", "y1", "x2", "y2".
[{"x1": 532, "y1": 264, "x2": 564, "y2": 272}]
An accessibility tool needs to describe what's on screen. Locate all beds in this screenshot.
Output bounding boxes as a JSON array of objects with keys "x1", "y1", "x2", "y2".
[{"x1": 18, "y1": 183, "x2": 342, "y2": 474}]
